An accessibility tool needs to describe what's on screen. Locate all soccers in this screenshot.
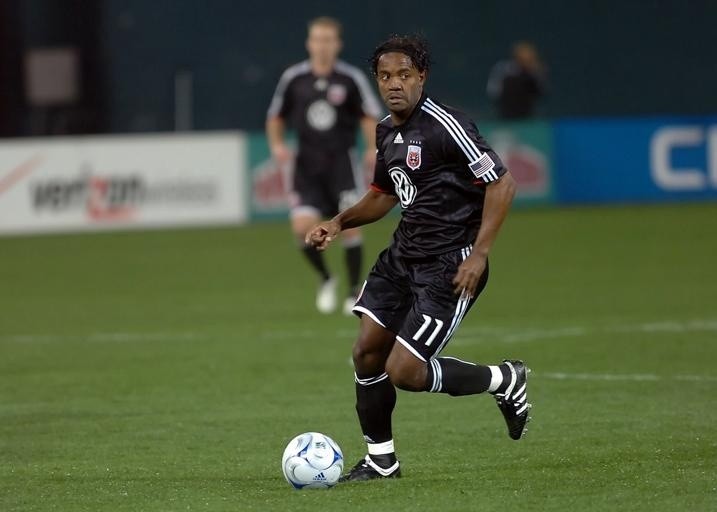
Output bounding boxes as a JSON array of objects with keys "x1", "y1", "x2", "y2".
[{"x1": 283, "y1": 433, "x2": 343, "y2": 491}]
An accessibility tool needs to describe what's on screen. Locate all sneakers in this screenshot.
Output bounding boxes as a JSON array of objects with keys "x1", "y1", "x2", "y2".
[
  {"x1": 493, "y1": 358, "x2": 532, "y2": 440},
  {"x1": 316, "y1": 277, "x2": 359, "y2": 316},
  {"x1": 338, "y1": 456, "x2": 401, "y2": 481}
]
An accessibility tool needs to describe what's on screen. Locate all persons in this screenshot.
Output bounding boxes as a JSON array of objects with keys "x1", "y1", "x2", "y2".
[
  {"x1": 266, "y1": 16, "x2": 384, "y2": 319},
  {"x1": 304, "y1": 33, "x2": 532, "y2": 485},
  {"x1": 488, "y1": 40, "x2": 545, "y2": 117}
]
